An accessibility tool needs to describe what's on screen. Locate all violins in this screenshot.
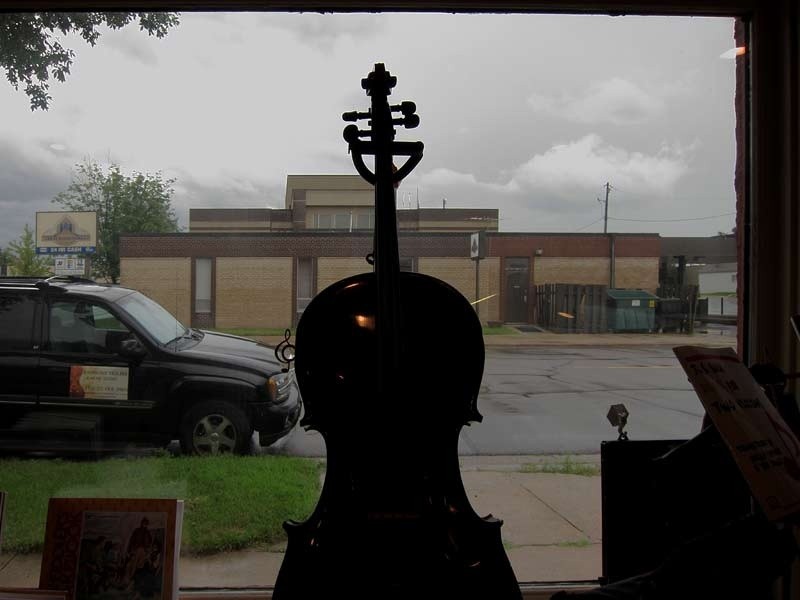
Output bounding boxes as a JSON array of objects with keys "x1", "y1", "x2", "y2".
[{"x1": 268, "y1": 63, "x2": 525, "y2": 600}]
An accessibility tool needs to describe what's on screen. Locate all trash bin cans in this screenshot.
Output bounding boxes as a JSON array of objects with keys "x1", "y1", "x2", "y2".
[{"x1": 604, "y1": 289, "x2": 658, "y2": 335}]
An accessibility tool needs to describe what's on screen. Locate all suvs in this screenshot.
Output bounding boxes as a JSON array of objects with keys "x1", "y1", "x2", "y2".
[{"x1": 0, "y1": 273, "x2": 303, "y2": 460}]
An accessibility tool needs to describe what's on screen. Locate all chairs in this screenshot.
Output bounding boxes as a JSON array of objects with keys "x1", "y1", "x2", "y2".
[{"x1": 72, "y1": 302, "x2": 96, "y2": 354}]
